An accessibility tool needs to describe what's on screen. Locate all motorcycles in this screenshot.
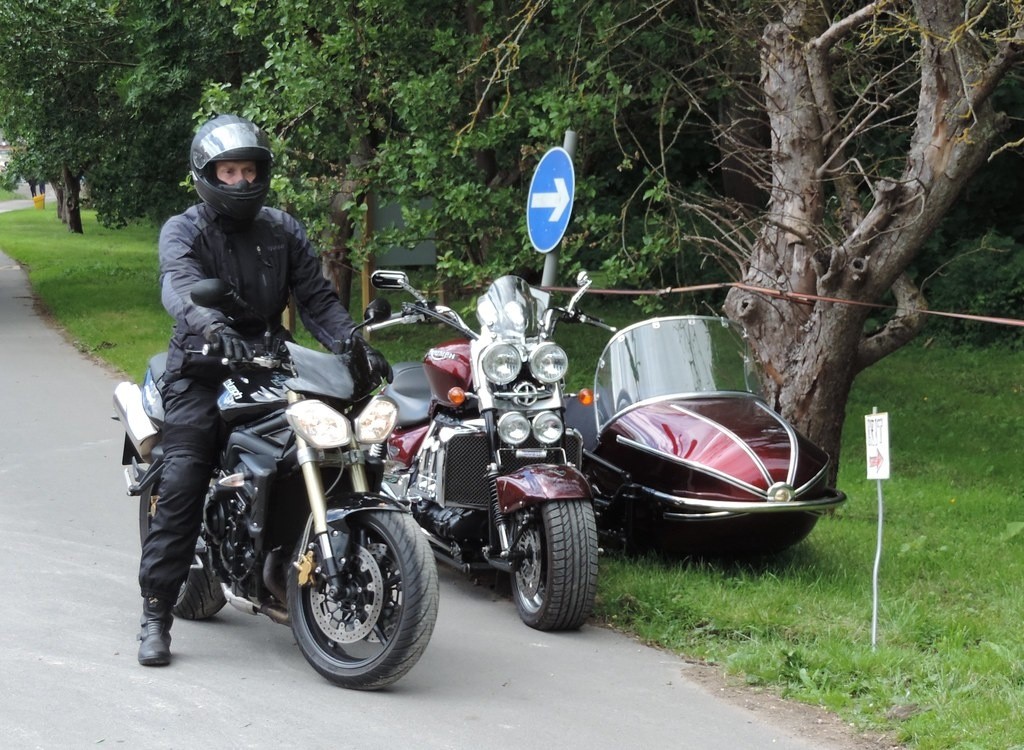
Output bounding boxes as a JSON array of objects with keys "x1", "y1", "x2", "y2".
[
  {"x1": 375, "y1": 269, "x2": 847, "y2": 634},
  {"x1": 111, "y1": 276, "x2": 440, "y2": 691}
]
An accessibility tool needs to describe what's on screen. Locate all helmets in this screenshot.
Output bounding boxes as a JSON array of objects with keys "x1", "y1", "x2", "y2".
[{"x1": 189, "y1": 115, "x2": 274, "y2": 220}]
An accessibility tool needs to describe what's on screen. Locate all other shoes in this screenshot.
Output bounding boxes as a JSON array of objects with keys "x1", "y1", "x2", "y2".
[{"x1": 136, "y1": 597, "x2": 173, "y2": 665}]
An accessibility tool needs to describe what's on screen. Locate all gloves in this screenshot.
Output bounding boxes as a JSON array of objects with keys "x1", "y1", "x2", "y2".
[
  {"x1": 343, "y1": 337, "x2": 394, "y2": 384},
  {"x1": 206, "y1": 322, "x2": 253, "y2": 363}
]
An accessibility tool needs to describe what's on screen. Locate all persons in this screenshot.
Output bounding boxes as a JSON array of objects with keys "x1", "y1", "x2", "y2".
[{"x1": 138, "y1": 114, "x2": 394, "y2": 665}]
